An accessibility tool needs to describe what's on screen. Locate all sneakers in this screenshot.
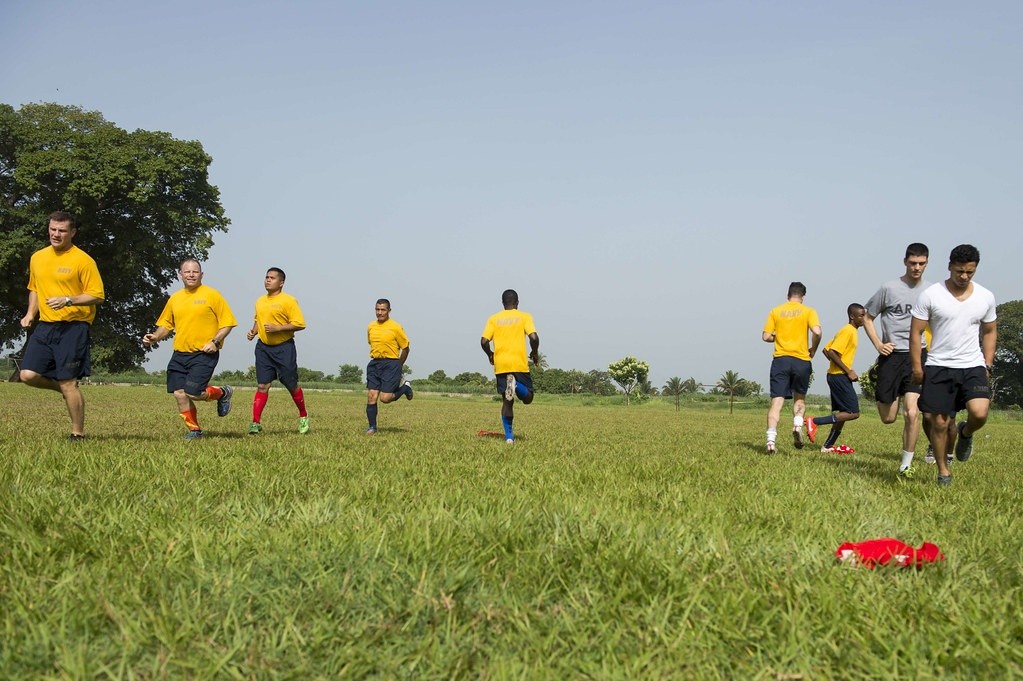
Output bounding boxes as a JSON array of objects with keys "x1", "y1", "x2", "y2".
[
  {"x1": 217, "y1": 384, "x2": 234, "y2": 417},
  {"x1": 946, "y1": 453, "x2": 954, "y2": 466},
  {"x1": 897, "y1": 467, "x2": 911, "y2": 483},
  {"x1": 925, "y1": 445, "x2": 936, "y2": 464},
  {"x1": 365, "y1": 427, "x2": 378, "y2": 435},
  {"x1": 955, "y1": 422, "x2": 973, "y2": 461},
  {"x1": 937, "y1": 475, "x2": 951, "y2": 488},
  {"x1": 767, "y1": 442, "x2": 776, "y2": 455},
  {"x1": 792, "y1": 427, "x2": 805, "y2": 449},
  {"x1": 187, "y1": 429, "x2": 203, "y2": 440},
  {"x1": 506, "y1": 375, "x2": 516, "y2": 402},
  {"x1": 247, "y1": 422, "x2": 262, "y2": 434},
  {"x1": 804, "y1": 416, "x2": 816, "y2": 443},
  {"x1": 404, "y1": 380, "x2": 414, "y2": 400},
  {"x1": 821, "y1": 445, "x2": 838, "y2": 453},
  {"x1": 298, "y1": 412, "x2": 312, "y2": 434}
]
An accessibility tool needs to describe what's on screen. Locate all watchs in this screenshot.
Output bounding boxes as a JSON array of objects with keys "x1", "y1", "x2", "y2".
[
  {"x1": 987, "y1": 365, "x2": 996, "y2": 374},
  {"x1": 212, "y1": 339, "x2": 220, "y2": 348},
  {"x1": 66, "y1": 296, "x2": 72, "y2": 307}
]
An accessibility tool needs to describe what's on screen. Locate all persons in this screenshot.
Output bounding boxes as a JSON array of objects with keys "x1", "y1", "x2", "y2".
[
  {"x1": 863, "y1": 243, "x2": 934, "y2": 478},
  {"x1": 804, "y1": 303, "x2": 865, "y2": 452},
  {"x1": 909, "y1": 245, "x2": 996, "y2": 488},
  {"x1": 366, "y1": 299, "x2": 413, "y2": 433},
  {"x1": 481, "y1": 289, "x2": 539, "y2": 442},
  {"x1": 762, "y1": 282, "x2": 822, "y2": 452},
  {"x1": 143, "y1": 259, "x2": 237, "y2": 438},
  {"x1": 21, "y1": 212, "x2": 104, "y2": 441},
  {"x1": 246, "y1": 268, "x2": 310, "y2": 434}
]
